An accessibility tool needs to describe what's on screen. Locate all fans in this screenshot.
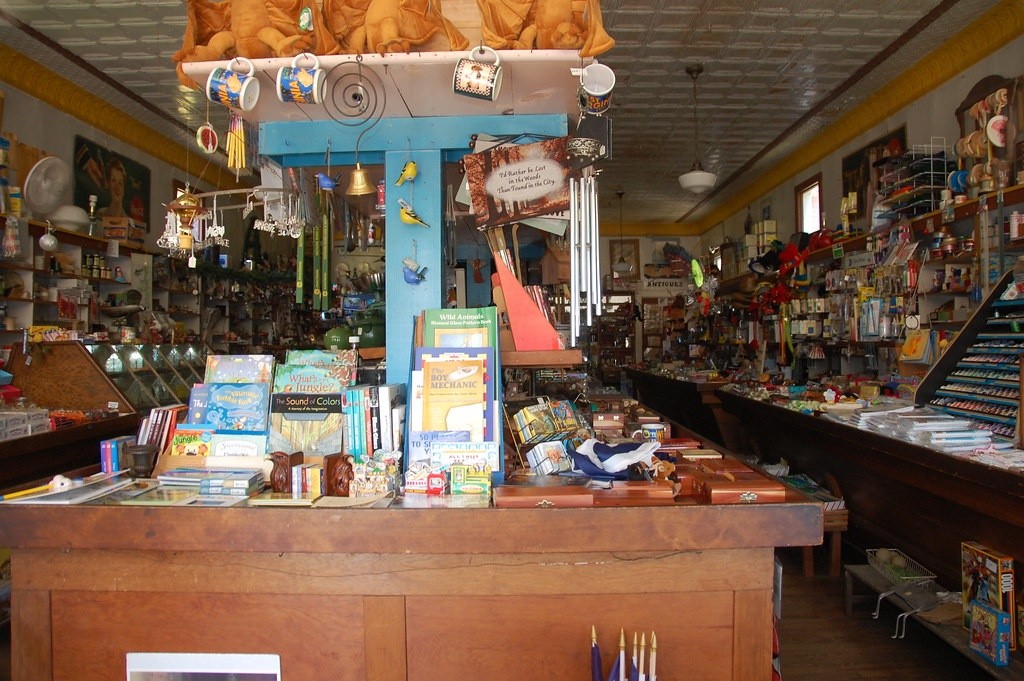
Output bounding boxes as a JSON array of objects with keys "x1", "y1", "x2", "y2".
[{"x1": 24, "y1": 156, "x2": 72, "y2": 216}]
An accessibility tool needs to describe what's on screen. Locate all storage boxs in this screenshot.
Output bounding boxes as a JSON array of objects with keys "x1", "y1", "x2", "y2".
[
  {"x1": 0, "y1": 358, "x2": 91, "y2": 440},
  {"x1": 960, "y1": 540, "x2": 1024, "y2": 667},
  {"x1": 219, "y1": 254, "x2": 233, "y2": 269},
  {"x1": 101, "y1": 216, "x2": 146, "y2": 247},
  {"x1": 764, "y1": 457, "x2": 846, "y2": 510},
  {"x1": 30, "y1": 318, "x2": 110, "y2": 344},
  {"x1": 403, "y1": 394, "x2": 785, "y2": 510},
  {"x1": 942, "y1": 255, "x2": 1014, "y2": 320},
  {"x1": 721, "y1": 219, "x2": 776, "y2": 280}
]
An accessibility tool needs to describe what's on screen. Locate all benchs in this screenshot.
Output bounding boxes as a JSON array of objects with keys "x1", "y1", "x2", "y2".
[{"x1": 843, "y1": 562, "x2": 1024, "y2": 681}]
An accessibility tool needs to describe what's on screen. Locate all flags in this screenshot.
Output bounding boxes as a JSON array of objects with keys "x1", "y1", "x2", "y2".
[{"x1": 592, "y1": 640, "x2": 659, "y2": 681}]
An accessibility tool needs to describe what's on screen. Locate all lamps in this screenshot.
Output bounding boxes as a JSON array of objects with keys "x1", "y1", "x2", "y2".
[
  {"x1": 165, "y1": 89, "x2": 208, "y2": 250},
  {"x1": 610, "y1": 189, "x2": 633, "y2": 272},
  {"x1": 676, "y1": 63, "x2": 718, "y2": 196}
]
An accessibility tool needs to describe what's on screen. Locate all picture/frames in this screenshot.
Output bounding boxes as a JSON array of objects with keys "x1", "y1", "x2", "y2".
[{"x1": 610, "y1": 238, "x2": 640, "y2": 280}]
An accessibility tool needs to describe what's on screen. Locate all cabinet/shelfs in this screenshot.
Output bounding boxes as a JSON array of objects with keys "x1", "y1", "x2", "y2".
[
  {"x1": 594, "y1": 321, "x2": 635, "y2": 383},
  {"x1": 678, "y1": 184, "x2": 1024, "y2": 383},
  {"x1": 874, "y1": 136, "x2": 957, "y2": 221},
  {"x1": 4, "y1": 341, "x2": 218, "y2": 418},
  {"x1": 913, "y1": 266, "x2": 1024, "y2": 448},
  {"x1": 0, "y1": 213, "x2": 293, "y2": 354}
]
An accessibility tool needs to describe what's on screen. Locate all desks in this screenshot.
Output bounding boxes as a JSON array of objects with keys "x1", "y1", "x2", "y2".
[
  {"x1": 0, "y1": 412, "x2": 138, "y2": 637},
  {"x1": 715, "y1": 382, "x2": 1024, "y2": 609},
  {"x1": 623, "y1": 366, "x2": 748, "y2": 452}
]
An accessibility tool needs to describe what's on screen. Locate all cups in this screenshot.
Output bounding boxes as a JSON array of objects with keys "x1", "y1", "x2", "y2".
[
  {"x1": 453, "y1": 46, "x2": 503, "y2": 102},
  {"x1": 205, "y1": 57, "x2": 260, "y2": 111},
  {"x1": 632, "y1": 423, "x2": 665, "y2": 444},
  {"x1": 275, "y1": 53, "x2": 326, "y2": 105},
  {"x1": 578, "y1": 58, "x2": 615, "y2": 114}
]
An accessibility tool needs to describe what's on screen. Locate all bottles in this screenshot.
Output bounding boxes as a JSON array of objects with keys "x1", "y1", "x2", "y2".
[
  {"x1": 353, "y1": 268, "x2": 357, "y2": 279},
  {"x1": 349, "y1": 337, "x2": 362, "y2": 367},
  {"x1": 879, "y1": 311, "x2": 890, "y2": 337},
  {"x1": 1010, "y1": 211, "x2": 1022, "y2": 238},
  {"x1": 891, "y1": 317, "x2": 898, "y2": 336},
  {"x1": 81, "y1": 195, "x2": 102, "y2": 238}
]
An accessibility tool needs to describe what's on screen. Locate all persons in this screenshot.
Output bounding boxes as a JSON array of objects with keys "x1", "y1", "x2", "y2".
[{"x1": 94, "y1": 156, "x2": 128, "y2": 220}]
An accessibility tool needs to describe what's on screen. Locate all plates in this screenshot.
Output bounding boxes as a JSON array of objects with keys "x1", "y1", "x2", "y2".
[
  {"x1": 336, "y1": 262, "x2": 350, "y2": 276},
  {"x1": 358, "y1": 262, "x2": 370, "y2": 274}
]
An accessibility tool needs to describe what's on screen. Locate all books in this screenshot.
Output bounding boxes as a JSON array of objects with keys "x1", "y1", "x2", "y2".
[{"x1": 0, "y1": 306, "x2": 581, "y2": 506}]
